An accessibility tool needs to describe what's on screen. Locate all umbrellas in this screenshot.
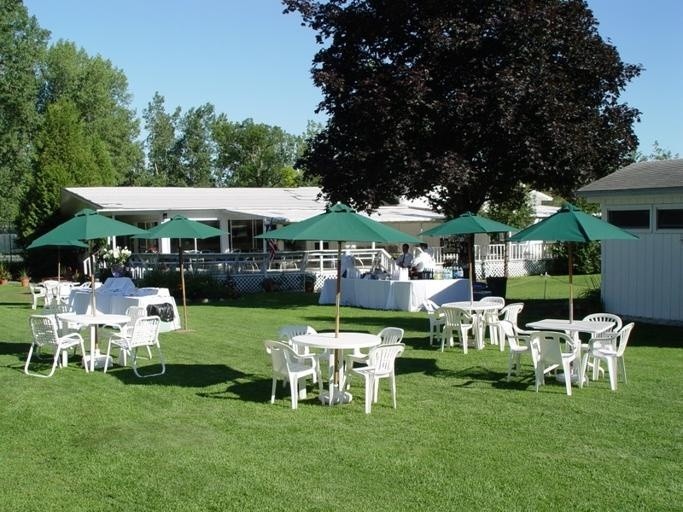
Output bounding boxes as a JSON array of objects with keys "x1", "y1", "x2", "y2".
[
  {"x1": 418, "y1": 211, "x2": 520, "y2": 306},
  {"x1": 252, "y1": 204, "x2": 426, "y2": 384},
  {"x1": 32, "y1": 208, "x2": 150, "y2": 357},
  {"x1": 129, "y1": 215, "x2": 231, "y2": 330},
  {"x1": 26, "y1": 240, "x2": 90, "y2": 282},
  {"x1": 505, "y1": 201, "x2": 642, "y2": 365}
]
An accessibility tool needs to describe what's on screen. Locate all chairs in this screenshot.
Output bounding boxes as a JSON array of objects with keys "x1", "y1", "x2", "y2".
[{"x1": 184, "y1": 248, "x2": 298, "y2": 270}]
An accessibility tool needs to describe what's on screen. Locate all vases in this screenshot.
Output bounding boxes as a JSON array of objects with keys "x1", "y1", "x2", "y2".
[{"x1": 110, "y1": 263, "x2": 124, "y2": 276}]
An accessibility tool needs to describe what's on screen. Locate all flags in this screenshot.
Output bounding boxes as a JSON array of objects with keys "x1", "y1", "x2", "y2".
[{"x1": 265, "y1": 217, "x2": 276, "y2": 262}]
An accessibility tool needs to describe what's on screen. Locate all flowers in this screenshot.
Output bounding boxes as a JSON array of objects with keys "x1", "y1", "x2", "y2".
[{"x1": 102, "y1": 245, "x2": 132, "y2": 263}]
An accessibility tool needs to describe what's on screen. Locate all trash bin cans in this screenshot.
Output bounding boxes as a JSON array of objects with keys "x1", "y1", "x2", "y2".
[
  {"x1": 486, "y1": 276, "x2": 507, "y2": 298},
  {"x1": 151, "y1": 304, "x2": 171, "y2": 333}
]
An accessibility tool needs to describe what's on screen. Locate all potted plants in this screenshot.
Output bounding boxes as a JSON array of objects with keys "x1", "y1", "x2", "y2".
[
  {"x1": 16, "y1": 268, "x2": 31, "y2": 287},
  {"x1": 0, "y1": 271, "x2": 12, "y2": 285}
]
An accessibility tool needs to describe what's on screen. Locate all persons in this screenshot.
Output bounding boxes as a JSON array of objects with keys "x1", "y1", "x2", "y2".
[
  {"x1": 410, "y1": 243, "x2": 433, "y2": 279},
  {"x1": 418, "y1": 242, "x2": 433, "y2": 257},
  {"x1": 394, "y1": 244, "x2": 413, "y2": 268}
]
